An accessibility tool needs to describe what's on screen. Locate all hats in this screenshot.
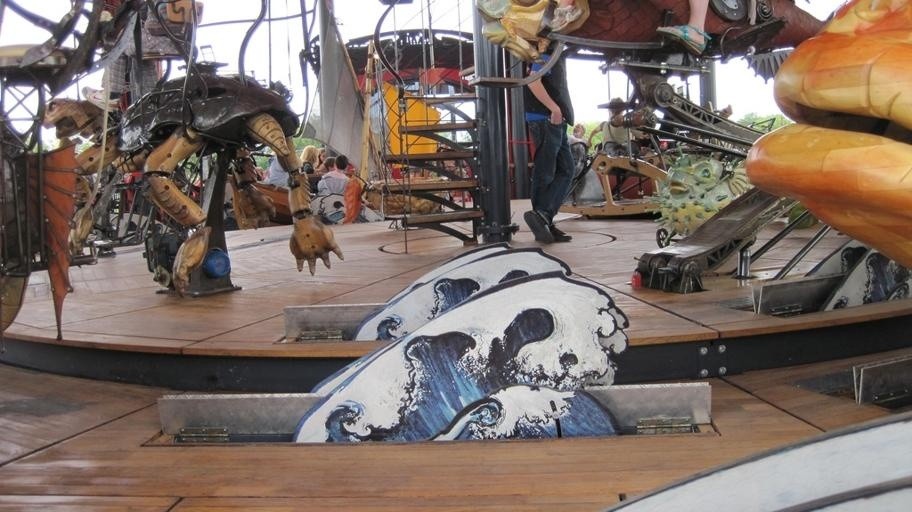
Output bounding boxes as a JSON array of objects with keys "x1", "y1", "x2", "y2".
[{"x1": 597, "y1": 97, "x2": 628, "y2": 109}]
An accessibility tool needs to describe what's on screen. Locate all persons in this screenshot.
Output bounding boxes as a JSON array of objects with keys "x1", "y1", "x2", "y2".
[
  {"x1": 83, "y1": 1, "x2": 167, "y2": 116},
  {"x1": 254, "y1": 146, "x2": 351, "y2": 198},
  {"x1": 587, "y1": 97, "x2": 636, "y2": 145},
  {"x1": 568, "y1": 124, "x2": 587, "y2": 144},
  {"x1": 658, "y1": 0, "x2": 711, "y2": 57},
  {"x1": 524, "y1": 43, "x2": 581, "y2": 247}
]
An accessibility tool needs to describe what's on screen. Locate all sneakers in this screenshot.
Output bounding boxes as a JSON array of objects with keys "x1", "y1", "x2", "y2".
[{"x1": 523, "y1": 210, "x2": 573, "y2": 244}]
